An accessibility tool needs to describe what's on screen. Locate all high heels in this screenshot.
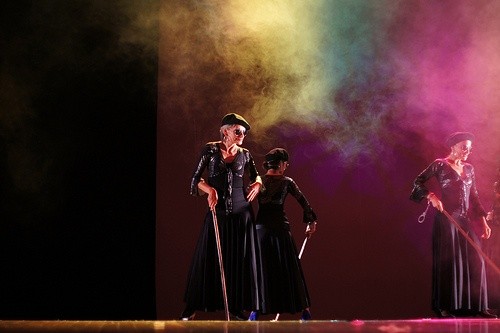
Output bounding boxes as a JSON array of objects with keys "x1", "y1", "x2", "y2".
[
  {"x1": 248, "y1": 310, "x2": 257, "y2": 321},
  {"x1": 178, "y1": 311, "x2": 196, "y2": 320},
  {"x1": 301, "y1": 309, "x2": 312, "y2": 320}
]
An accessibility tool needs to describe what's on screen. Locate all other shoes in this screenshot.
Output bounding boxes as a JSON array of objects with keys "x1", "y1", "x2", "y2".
[
  {"x1": 473, "y1": 310, "x2": 497, "y2": 318},
  {"x1": 434, "y1": 309, "x2": 455, "y2": 319}
]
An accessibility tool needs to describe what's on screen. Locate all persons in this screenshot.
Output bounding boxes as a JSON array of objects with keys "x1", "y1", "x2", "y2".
[
  {"x1": 409, "y1": 131, "x2": 497, "y2": 318},
  {"x1": 247, "y1": 147, "x2": 319, "y2": 320},
  {"x1": 177, "y1": 112, "x2": 266, "y2": 320}
]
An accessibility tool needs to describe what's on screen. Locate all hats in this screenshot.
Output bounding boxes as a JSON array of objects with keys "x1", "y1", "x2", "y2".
[
  {"x1": 448, "y1": 131, "x2": 474, "y2": 146},
  {"x1": 264, "y1": 148, "x2": 288, "y2": 161},
  {"x1": 222, "y1": 113, "x2": 251, "y2": 130}
]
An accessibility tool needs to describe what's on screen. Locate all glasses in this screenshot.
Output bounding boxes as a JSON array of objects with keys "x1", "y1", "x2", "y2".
[
  {"x1": 284, "y1": 162, "x2": 290, "y2": 167},
  {"x1": 454, "y1": 144, "x2": 473, "y2": 152},
  {"x1": 227, "y1": 128, "x2": 248, "y2": 136}
]
